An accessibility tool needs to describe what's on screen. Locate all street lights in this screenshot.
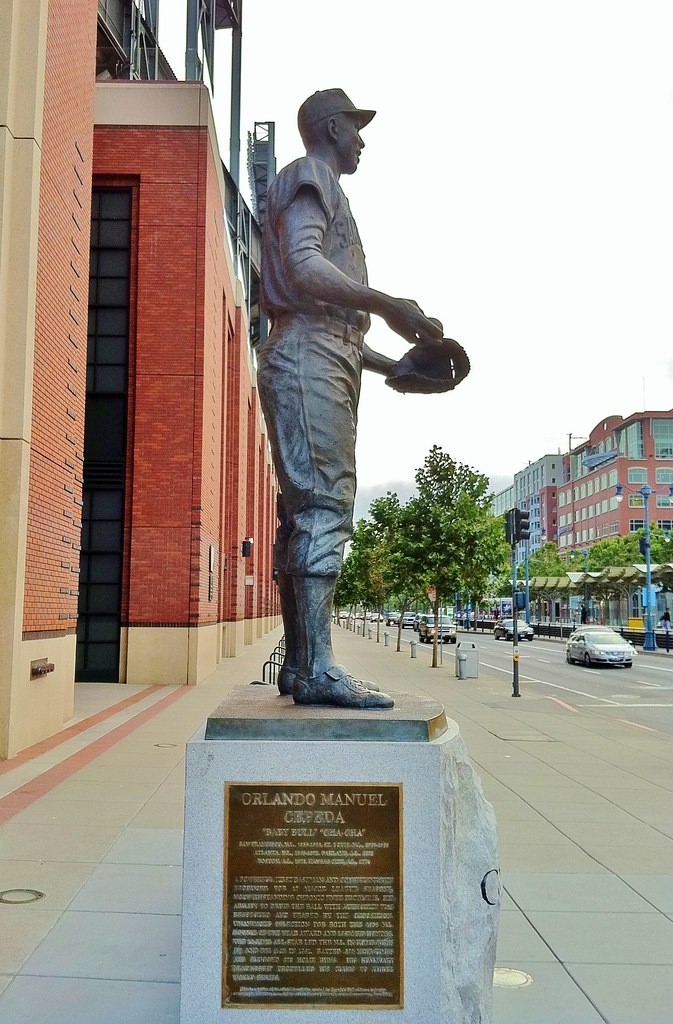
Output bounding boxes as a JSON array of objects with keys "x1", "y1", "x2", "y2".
[
  {"x1": 615, "y1": 483, "x2": 673, "y2": 650},
  {"x1": 570, "y1": 549, "x2": 589, "y2": 623}
]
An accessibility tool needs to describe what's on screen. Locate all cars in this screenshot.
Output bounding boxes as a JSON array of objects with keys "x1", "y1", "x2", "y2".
[
  {"x1": 494, "y1": 619, "x2": 534, "y2": 642},
  {"x1": 566, "y1": 625, "x2": 634, "y2": 668},
  {"x1": 370, "y1": 613, "x2": 383, "y2": 622},
  {"x1": 339, "y1": 611, "x2": 371, "y2": 620}
]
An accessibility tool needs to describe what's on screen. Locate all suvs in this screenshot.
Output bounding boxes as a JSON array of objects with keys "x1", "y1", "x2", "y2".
[
  {"x1": 398, "y1": 612, "x2": 417, "y2": 628},
  {"x1": 418, "y1": 615, "x2": 457, "y2": 644},
  {"x1": 413, "y1": 612, "x2": 435, "y2": 631},
  {"x1": 385, "y1": 612, "x2": 401, "y2": 626}
]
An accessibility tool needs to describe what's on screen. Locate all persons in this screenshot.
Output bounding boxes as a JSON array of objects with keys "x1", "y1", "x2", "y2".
[
  {"x1": 256, "y1": 87, "x2": 443, "y2": 708},
  {"x1": 481, "y1": 610, "x2": 485, "y2": 619},
  {"x1": 664, "y1": 608, "x2": 671, "y2": 629},
  {"x1": 581, "y1": 605, "x2": 587, "y2": 624},
  {"x1": 494, "y1": 609, "x2": 499, "y2": 620}
]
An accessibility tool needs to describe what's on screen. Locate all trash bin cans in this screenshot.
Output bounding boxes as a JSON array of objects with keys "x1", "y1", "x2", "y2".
[
  {"x1": 346, "y1": 618, "x2": 353, "y2": 629},
  {"x1": 360, "y1": 621, "x2": 370, "y2": 635},
  {"x1": 455, "y1": 641, "x2": 479, "y2": 677}
]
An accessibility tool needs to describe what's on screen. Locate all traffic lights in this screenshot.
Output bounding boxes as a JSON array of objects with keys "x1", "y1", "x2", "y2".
[{"x1": 516, "y1": 509, "x2": 530, "y2": 541}]
